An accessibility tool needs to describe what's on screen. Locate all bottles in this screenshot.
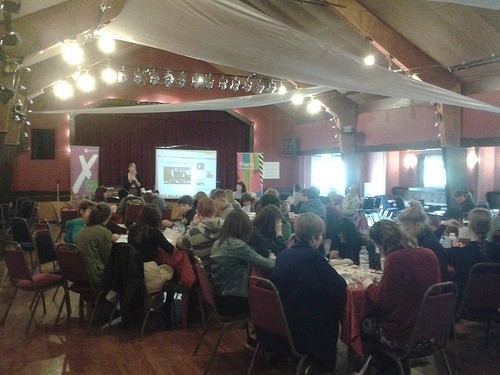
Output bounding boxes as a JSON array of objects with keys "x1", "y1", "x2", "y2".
[
  {"x1": 359, "y1": 246, "x2": 369, "y2": 274},
  {"x1": 380, "y1": 250, "x2": 386, "y2": 271}
]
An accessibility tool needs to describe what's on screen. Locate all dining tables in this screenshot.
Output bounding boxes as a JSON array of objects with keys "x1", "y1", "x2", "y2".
[{"x1": 340, "y1": 282, "x2": 383, "y2": 375}]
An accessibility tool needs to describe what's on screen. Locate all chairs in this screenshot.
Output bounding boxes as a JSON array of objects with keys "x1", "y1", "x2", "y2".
[
  {"x1": 193, "y1": 255, "x2": 249, "y2": 375},
  {"x1": 357, "y1": 209, "x2": 500, "y2": 375},
  {"x1": 0, "y1": 198, "x2": 207, "y2": 342},
  {"x1": 245, "y1": 276, "x2": 310, "y2": 375}
]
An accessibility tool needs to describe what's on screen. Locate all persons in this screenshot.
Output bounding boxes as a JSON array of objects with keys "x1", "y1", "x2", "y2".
[
  {"x1": 167, "y1": 169, "x2": 188, "y2": 185},
  {"x1": 122, "y1": 162, "x2": 143, "y2": 197},
  {"x1": 64, "y1": 182, "x2": 500, "y2": 375}
]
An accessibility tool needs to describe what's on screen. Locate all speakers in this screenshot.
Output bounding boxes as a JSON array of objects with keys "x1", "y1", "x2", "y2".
[{"x1": 284, "y1": 138, "x2": 298, "y2": 155}]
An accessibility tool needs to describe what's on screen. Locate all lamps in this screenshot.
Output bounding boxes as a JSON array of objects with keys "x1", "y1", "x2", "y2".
[{"x1": 119, "y1": 65, "x2": 277, "y2": 94}]
